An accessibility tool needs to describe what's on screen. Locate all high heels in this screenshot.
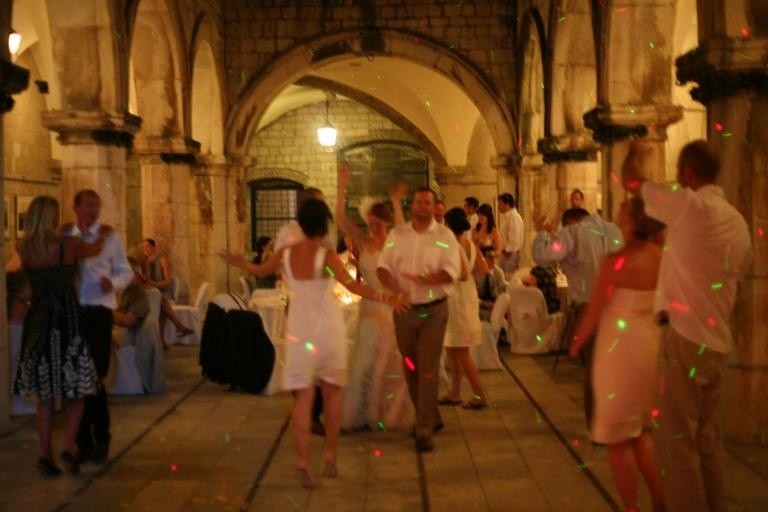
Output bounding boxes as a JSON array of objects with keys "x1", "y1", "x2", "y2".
[
  {"x1": 296, "y1": 459, "x2": 320, "y2": 488},
  {"x1": 37, "y1": 457, "x2": 62, "y2": 480},
  {"x1": 60, "y1": 450, "x2": 81, "y2": 475},
  {"x1": 321, "y1": 451, "x2": 337, "y2": 479}
]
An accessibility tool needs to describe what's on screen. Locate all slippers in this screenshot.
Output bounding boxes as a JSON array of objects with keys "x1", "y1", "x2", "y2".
[
  {"x1": 437, "y1": 395, "x2": 463, "y2": 406},
  {"x1": 462, "y1": 395, "x2": 488, "y2": 409},
  {"x1": 177, "y1": 329, "x2": 194, "y2": 337}
]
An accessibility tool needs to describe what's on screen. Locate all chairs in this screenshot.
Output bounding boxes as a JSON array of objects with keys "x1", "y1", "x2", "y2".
[
  {"x1": 198, "y1": 293, "x2": 247, "y2": 384},
  {"x1": 511, "y1": 286, "x2": 565, "y2": 355},
  {"x1": 109, "y1": 289, "x2": 161, "y2": 395},
  {"x1": 473, "y1": 292, "x2": 513, "y2": 371},
  {"x1": 228, "y1": 309, "x2": 279, "y2": 396},
  {"x1": 162, "y1": 281, "x2": 209, "y2": 344},
  {"x1": 237, "y1": 275, "x2": 253, "y2": 307}
]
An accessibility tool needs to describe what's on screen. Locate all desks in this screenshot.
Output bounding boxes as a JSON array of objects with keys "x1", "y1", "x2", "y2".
[
  {"x1": 505, "y1": 273, "x2": 570, "y2": 313},
  {"x1": 250, "y1": 288, "x2": 285, "y2": 338}
]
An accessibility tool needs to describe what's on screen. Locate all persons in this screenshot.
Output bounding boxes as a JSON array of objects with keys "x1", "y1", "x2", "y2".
[
  {"x1": 16, "y1": 195, "x2": 114, "y2": 477},
  {"x1": 376, "y1": 187, "x2": 461, "y2": 451},
  {"x1": 274, "y1": 187, "x2": 325, "y2": 253},
  {"x1": 254, "y1": 236, "x2": 283, "y2": 288},
  {"x1": 619, "y1": 140, "x2": 752, "y2": 512},
  {"x1": 218, "y1": 198, "x2": 412, "y2": 488},
  {"x1": 571, "y1": 198, "x2": 664, "y2": 511},
  {"x1": 113, "y1": 236, "x2": 195, "y2": 350},
  {"x1": 436, "y1": 192, "x2": 591, "y2": 432},
  {"x1": 337, "y1": 161, "x2": 418, "y2": 432},
  {"x1": 523, "y1": 207, "x2": 623, "y2": 446},
  {"x1": 7, "y1": 270, "x2": 32, "y2": 416},
  {"x1": 65, "y1": 189, "x2": 134, "y2": 465}
]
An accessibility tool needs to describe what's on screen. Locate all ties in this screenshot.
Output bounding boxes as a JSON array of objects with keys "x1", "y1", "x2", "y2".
[{"x1": 483, "y1": 274, "x2": 492, "y2": 301}]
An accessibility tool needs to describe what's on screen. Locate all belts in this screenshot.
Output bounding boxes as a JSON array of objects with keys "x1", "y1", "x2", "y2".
[{"x1": 408, "y1": 295, "x2": 448, "y2": 311}]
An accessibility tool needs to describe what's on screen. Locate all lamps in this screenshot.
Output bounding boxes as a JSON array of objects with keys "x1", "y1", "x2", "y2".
[{"x1": 318, "y1": 100, "x2": 338, "y2": 150}]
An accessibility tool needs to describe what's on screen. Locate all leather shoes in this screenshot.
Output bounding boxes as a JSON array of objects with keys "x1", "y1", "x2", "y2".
[
  {"x1": 415, "y1": 435, "x2": 434, "y2": 452},
  {"x1": 411, "y1": 421, "x2": 445, "y2": 437}
]
